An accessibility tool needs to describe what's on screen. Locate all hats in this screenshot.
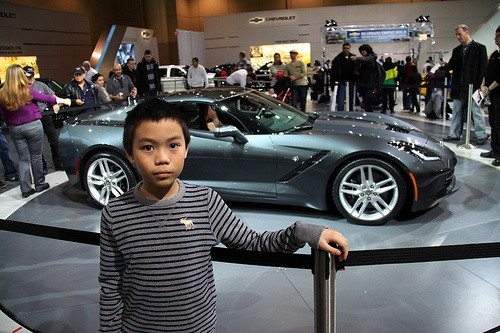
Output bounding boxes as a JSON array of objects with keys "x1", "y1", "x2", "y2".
[
  {"x1": 74, "y1": 67, "x2": 85, "y2": 73},
  {"x1": 23, "y1": 66, "x2": 34, "y2": 76},
  {"x1": 290, "y1": 50, "x2": 298, "y2": 54}
]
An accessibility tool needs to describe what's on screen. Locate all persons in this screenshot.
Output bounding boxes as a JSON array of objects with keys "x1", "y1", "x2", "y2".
[
  {"x1": 330, "y1": 42, "x2": 452, "y2": 119},
  {"x1": 187, "y1": 56, "x2": 210, "y2": 89},
  {"x1": 264, "y1": 47, "x2": 330, "y2": 113},
  {"x1": 224, "y1": 50, "x2": 253, "y2": 87},
  {"x1": 121, "y1": 49, "x2": 164, "y2": 97},
  {"x1": 1, "y1": 62, "x2": 85, "y2": 199},
  {"x1": 438, "y1": 23, "x2": 500, "y2": 165},
  {"x1": 97, "y1": 94, "x2": 351, "y2": 333},
  {"x1": 81, "y1": 58, "x2": 115, "y2": 111},
  {"x1": 106, "y1": 63, "x2": 138, "y2": 106},
  {"x1": 56, "y1": 66, "x2": 101, "y2": 114}
]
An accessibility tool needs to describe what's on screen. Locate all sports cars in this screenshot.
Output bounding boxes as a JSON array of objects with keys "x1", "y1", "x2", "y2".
[{"x1": 56, "y1": 85, "x2": 462, "y2": 226}]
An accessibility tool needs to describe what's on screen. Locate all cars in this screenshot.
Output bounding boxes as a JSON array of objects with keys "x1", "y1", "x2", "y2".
[
  {"x1": 35, "y1": 78, "x2": 63, "y2": 97},
  {"x1": 253, "y1": 61, "x2": 275, "y2": 90},
  {"x1": 159, "y1": 64, "x2": 216, "y2": 93}
]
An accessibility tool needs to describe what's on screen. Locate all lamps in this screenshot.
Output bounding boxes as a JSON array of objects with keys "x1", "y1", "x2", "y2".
[
  {"x1": 324, "y1": 19, "x2": 337, "y2": 27},
  {"x1": 415, "y1": 15, "x2": 430, "y2": 23}
]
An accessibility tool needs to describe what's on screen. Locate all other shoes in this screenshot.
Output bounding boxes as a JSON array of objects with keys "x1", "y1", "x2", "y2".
[
  {"x1": 5, "y1": 176, "x2": 19, "y2": 181},
  {"x1": 480, "y1": 152, "x2": 495, "y2": 157},
  {"x1": 23, "y1": 188, "x2": 36, "y2": 197},
  {"x1": 44, "y1": 169, "x2": 48, "y2": 175},
  {"x1": 0, "y1": 184, "x2": 7, "y2": 188},
  {"x1": 55, "y1": 166, "x2": 64, "y2": 171},
  {"x1": 443, "y1": 136, "x2": 456, "y2": 141},
  {"x1": 477, "y1": 137, "x2": 486, "y2": 145},
  {"x1": 37, "y1": 183, "x2": 50, "y2": 192},
  {"x1": 492, "y1": 159, "x2": 500, "y2": 166}
]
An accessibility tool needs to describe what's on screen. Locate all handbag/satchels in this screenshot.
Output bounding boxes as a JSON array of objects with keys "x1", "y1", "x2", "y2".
[{"x1": 374, "y1": 61, "x2": 386, "y2": 83}]
